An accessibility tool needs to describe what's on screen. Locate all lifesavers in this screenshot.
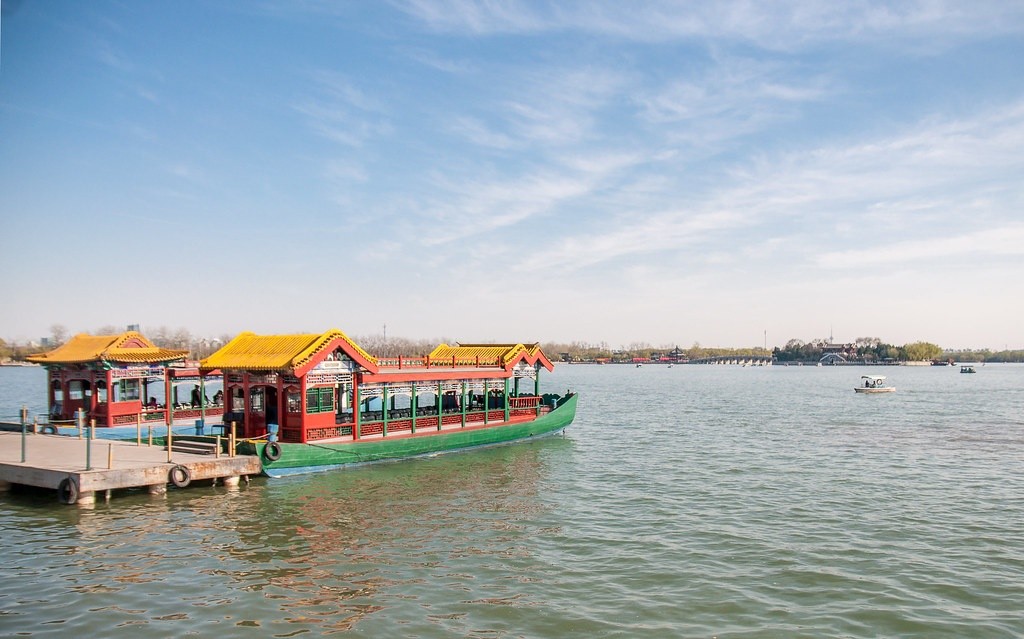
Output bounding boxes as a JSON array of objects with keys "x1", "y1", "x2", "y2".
[
  {"x1": 264, "y1": 441, "x2": 282, "y2": 461},
  {"x1": 170, "y1": 465, "x2": 191, "y2": 487},
  {"x1": 57, "y1": 477, "x2": 77, "y2": 504}
]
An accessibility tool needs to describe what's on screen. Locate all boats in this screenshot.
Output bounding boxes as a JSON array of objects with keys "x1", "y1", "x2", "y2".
[
  {"x1": 634, "y1": 364, "x2": 643, "y2": 368},
  {"x1": 0, "y1": 360, "x2": 41, "y2": 367},
  {"x1": 960, "y1": 366, "x2": 976, "y2": 373},
  {"x1": 0, "y1": 330, "x2": 224, "y2": 440},
  {"x1": 854, "y1": 375, "x2": 895, "y2": 393},
  {"x1": 930, "y1": 358, "x2": 957, "y2": 366},
  {"x1": 162, "y1": 331, "x2": 578, "y2": 479},
  {"x1": 668, "y1": 364, "x2": 675, "y2": 368}
]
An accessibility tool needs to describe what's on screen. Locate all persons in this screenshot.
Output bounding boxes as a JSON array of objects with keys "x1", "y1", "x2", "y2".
[
  {"x1": 191, "y1": 385, "x2": 202, "y2": 408},
  {"x1": 872, "y1": 381, "x2": 876, "y2": 386},
  {"x1": 213, "y1": 390, "x2": 223, "y2": 404},
  {"x1": 146, "y1": 397, "x2": 157, "y2": 410},
  {"x1": 866, "y1": 380, "x2": 870, "y2": 387}
]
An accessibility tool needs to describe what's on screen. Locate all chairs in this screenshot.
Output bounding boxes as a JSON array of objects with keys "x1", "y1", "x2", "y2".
[
  {"x1": 141, "y1": 401, "x2": 224, "y2": 414},
  {"x1": 335, "y1": 406, "x2": 475, "y2": 424}
]
